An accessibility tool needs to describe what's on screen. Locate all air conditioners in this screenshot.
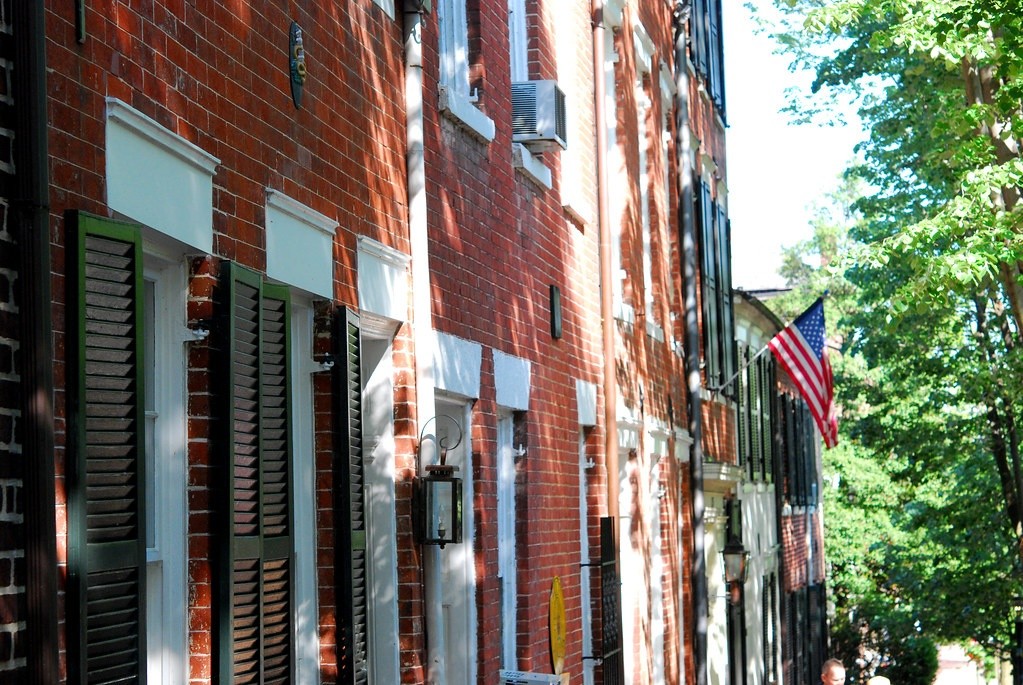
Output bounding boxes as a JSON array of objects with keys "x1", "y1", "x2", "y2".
[{"x1": 510, "y1": 79, "x2": 569, "y2": 156}]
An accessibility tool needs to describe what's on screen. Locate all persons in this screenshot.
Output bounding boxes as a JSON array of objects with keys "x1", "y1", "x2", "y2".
[
  {"x1": 866, "y1": 676, "x2": 890, "y2": 685},
  {"x1": 821, "y1": 658, "x2": 845, "y2": 685}
]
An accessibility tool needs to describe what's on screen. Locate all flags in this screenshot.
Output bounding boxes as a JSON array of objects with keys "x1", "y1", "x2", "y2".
[{"x1": 769, "y1": 299, "x2": 840, "y2": 448}]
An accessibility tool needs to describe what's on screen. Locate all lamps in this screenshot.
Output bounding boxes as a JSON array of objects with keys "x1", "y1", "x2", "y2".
[
  {"x1": 714, "y1": 530, "x2": 748, "y2": 603},
  {"x1": 413, "y1": 414, "x2": 464, "y2": 550}
]
